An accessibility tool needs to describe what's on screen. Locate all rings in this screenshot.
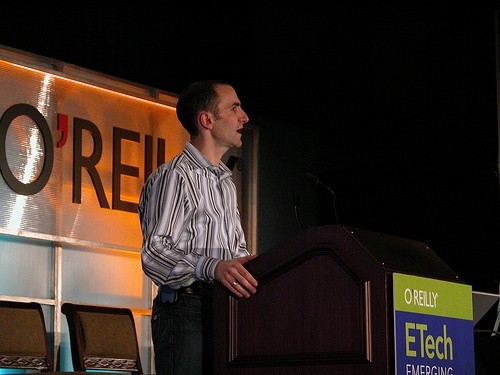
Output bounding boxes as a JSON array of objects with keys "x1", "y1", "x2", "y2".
[{"x1": 231, "y1": 281, "x2": 237, "y2": 286}]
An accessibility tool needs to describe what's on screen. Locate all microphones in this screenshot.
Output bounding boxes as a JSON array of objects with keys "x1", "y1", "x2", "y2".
[
  {"x1": 303, "y1": 172, "x2": 339, "y2": 225},
  {"x1": 292, "y1": 193, "x2": 303, "y2": 232}
]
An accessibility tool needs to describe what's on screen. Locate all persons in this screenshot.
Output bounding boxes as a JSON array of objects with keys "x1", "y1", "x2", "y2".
[{"x1": 138, "y1": 79, "x2": 259, "y2": 375}]
